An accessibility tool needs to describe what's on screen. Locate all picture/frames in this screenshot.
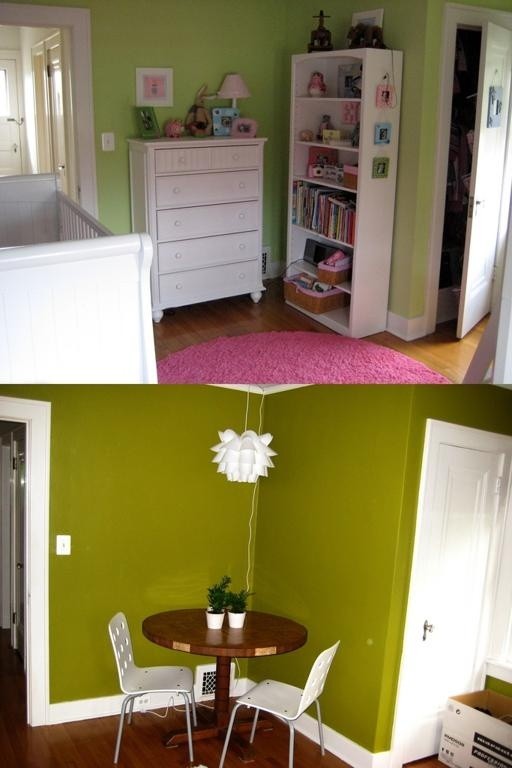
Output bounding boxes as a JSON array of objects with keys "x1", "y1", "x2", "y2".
[
  {"x1": 232, "y1": 118, "x2": 264, "y2": 135},
  {"x1": 130, "y1": 107, "x2": 162, "y2": 137},
  {"x1": 134, "y1": 66, "x2": 174, "y2": 108},
  {"x1": 211, "y1": 107, "x2": 241, "y2": 134},
  {"x1": 351, "y1": 7, "x2": 385, "y2": 45}
]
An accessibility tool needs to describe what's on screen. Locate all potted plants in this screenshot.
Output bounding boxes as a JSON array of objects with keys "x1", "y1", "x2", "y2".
[
  {"x1": 203, "y1": 572, "x2": 233, "y2": 626},
  {"x1": 227, "y1": 583, "x2": 258, "y2": 626}
]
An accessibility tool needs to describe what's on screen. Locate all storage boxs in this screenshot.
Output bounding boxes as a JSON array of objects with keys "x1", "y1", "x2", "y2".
[{"x1": 438, "y1": 687, "x2": 512, "y2": 767}]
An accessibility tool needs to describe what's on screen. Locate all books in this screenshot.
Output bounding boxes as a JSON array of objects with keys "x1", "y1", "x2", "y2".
[
  {"x1": 291, "y1": 179, "x2": 357, "y2": 248},
  {"x1": 309, "y1": 147, "x2": 337, "y2": 178}
]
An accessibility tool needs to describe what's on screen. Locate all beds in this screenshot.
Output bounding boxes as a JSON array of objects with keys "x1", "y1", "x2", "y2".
[{"x1": 0, "y1": 172, "x2": 157, "y2": 383}]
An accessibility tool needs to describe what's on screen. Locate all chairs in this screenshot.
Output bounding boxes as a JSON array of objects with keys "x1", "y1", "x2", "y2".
[
  {"x1": 216, "y1": 637, "x2": 343, "y2": 767},
  {"x1": 106, "y1": 609, "x2": 202, "y2": 763}
]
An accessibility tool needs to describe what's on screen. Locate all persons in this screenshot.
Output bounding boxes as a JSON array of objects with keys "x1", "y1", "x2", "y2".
[{"x1": 186, "y1": 83, "x2": 217, "y2": 139}]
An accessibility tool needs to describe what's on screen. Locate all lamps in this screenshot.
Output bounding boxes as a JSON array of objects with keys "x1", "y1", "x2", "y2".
[
  {"x1": 217, "y1": 71, "x2": 253, "y2": 111},
  {"x1": 212, "y1": 387, "x2": 276, "y2": 483}
]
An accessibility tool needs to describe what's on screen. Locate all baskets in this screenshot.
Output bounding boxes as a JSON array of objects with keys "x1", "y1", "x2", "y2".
[
  {"x1": 342, "y1": 164, "x2": 358, "y2": 190},
  {"x1": 316, "y1": 267, "x2": 352, "y2": 285},
  {"x1": 282, "y1": 272, "x2": 349, "y2": 315}
]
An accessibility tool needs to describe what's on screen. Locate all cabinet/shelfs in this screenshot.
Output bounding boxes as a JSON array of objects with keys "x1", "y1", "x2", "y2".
[
  {"x1": 282, "y1": 50, "x2": 406, "y2": 335},
  {"x1": 124, "y1": 136, "x2": 270, "y2": 322}
]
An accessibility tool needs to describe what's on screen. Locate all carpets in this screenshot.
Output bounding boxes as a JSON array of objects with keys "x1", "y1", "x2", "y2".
[{"x1": 153, "y1": 329, "x2": 460, "y2": 385}]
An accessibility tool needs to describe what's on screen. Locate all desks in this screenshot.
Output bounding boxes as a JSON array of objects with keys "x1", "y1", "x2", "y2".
[{"x1": 143, "y1": 607, "x2": 306, "y2": 762}]
